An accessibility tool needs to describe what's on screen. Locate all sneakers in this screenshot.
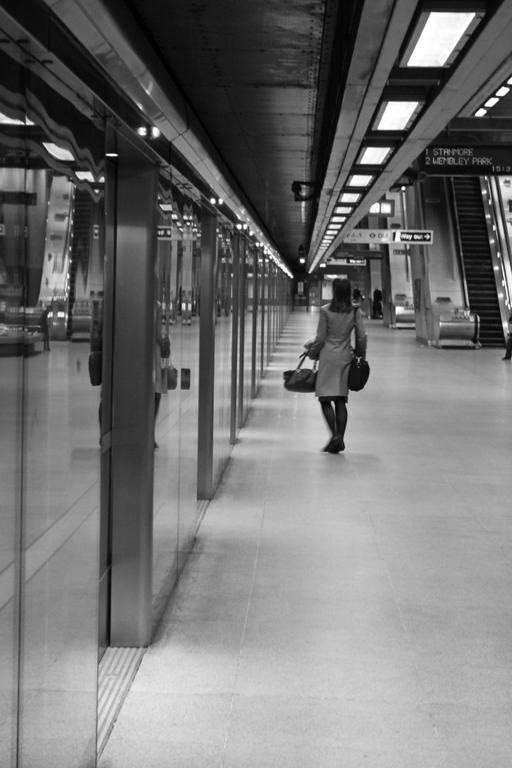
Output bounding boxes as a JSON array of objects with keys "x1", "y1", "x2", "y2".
[{"x1": 322, "y1": 433, "x2": 346, "y2": 453}]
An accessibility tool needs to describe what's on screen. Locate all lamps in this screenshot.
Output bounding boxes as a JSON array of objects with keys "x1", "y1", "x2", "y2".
[{"x1": 298, "y1": 245, "x2": 308, "y2": 264}]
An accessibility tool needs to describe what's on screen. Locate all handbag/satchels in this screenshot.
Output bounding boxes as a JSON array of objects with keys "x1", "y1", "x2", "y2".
[
  {"x1": 87, "y1": 348, "x2": 101, "y2": 386},
  {"x1": 281, "y1": 349, "x2": 319, "y2": 392},
  {"x1": 347, "y1": 349, "x2": 370, "y2": 391},
  {"x1": 160, "y1": 354, "x2": 178, "y2": 390}
]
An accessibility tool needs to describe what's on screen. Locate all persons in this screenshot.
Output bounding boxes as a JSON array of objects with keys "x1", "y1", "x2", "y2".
[
  {"x1": 502, "y1": 307, "x2": 512, "y2": 361},
  {"x1": 147, "y1": 277, "x2": 168, "y2": 449},
  {"x1": 351, "y1": 282, "x2": 366, "y2": 303},
  {"x1": 304, "y1": 277, "x2": 369, "y2": 456}
]
